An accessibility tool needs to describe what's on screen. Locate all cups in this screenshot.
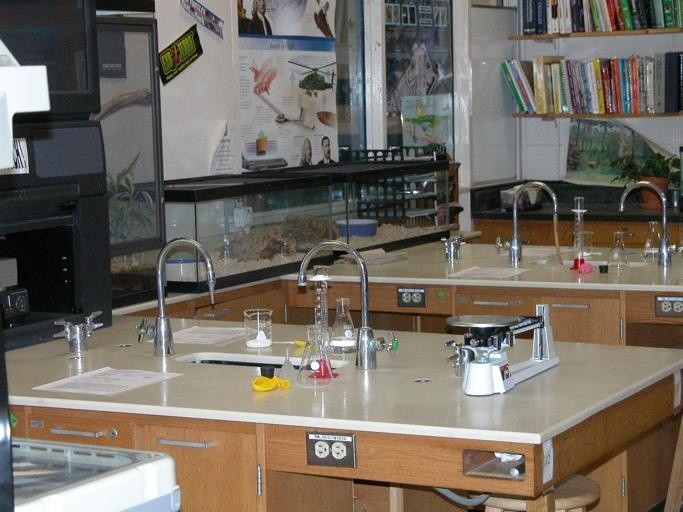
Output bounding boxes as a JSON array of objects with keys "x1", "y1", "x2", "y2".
[
  {"x1": 577, "y1": 230, "x2": 594, "y2": 258},
  {"x1": 241, "y1": 306, "x2": 272, "y2": 349}
]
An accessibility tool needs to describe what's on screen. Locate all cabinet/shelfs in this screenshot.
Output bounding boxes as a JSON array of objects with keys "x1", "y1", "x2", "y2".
[
  {"x1": 582, "y1": 411, "x2": 681, "y2": 511},
  {"x1": 24, "y1": 403, "x2": 357, "y2": 512},
  {"x1": 470, "y1": 210, "x2": 681, "y2": 248},
  {"x1": 496, "y1": 26, "x2": 683, "y2": 121},
  {"x1": 451, "y1": 286, "x2": 626, "y2": 348}
]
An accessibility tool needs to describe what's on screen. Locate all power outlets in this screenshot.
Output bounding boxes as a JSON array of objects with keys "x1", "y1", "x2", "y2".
[
  {"x1": 652, "y1": 293, "x2": 683, "y2": 320},
  {"x1": 395, "y1": 286, "x2": 426, "y2": 309},
  {"x1": 302, "y1": 429, "x2": 357, "y2": 471}
]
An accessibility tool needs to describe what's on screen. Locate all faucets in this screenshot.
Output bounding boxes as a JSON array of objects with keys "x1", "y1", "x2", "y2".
[
  {"x1": 618, "y1": 180, "x2": 679, "y2": 267},
  {"x1": 495, "y1": 181, "x2": 558, "y2": 263},
  {"x1": 134, "y1": 237, "x2": 216, "y2": 358},
  {"x1": 297, "y1": 239, "x2": 399, "y2": 370}
]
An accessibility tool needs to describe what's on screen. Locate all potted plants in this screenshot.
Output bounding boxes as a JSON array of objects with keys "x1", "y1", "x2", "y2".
[{"x1": 605, "y1": 145, "x2": 681, "y2": 213}]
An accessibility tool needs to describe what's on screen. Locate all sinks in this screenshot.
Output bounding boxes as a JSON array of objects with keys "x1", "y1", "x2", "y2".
[
  {"x1": 533, "y1": 260, "x2": 651, "y2": 268},
  {"x1": 174, "y1": 352, "x2": 351, "y2": 370}
]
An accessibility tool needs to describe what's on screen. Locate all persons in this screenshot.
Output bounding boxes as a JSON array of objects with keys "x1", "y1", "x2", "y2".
[
  {"x1": 300, "y1": 137, "x2": 314, "y2": 168},
  {"x1": 317, "y1": 136, "x2": 337, "y2": 165},
  {"x1": 237, "y1": 0, "x2": 273, "y2": 36}
]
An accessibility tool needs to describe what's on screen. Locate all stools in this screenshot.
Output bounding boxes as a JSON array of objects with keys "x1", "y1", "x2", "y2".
[{"x1": 464, "y1": 470, "x2": 602, "y2": 511}]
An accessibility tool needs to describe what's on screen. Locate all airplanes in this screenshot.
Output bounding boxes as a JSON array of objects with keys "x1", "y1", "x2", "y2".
[
  {"x1": 241, "y1": 151, "x2": 288, "y2": 170},
  {"x1": 260, "y1": 95, "x2": 315, "y2": 131}
]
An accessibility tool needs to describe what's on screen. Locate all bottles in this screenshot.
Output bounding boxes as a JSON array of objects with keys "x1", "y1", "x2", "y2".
[
  {"x1": 275, "y1": 265, "x2": 357, "y2": 390},
  {"x1": 639, "y1": 220, "x2": 664, "y2": 259},
  {"x1": 607, "y1": 231, "x2": 631, "y2": 272}
]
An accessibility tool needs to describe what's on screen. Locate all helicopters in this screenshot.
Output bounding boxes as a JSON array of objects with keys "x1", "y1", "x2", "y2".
[{"x1": 285, "y1": 59, "x2": 336, "y2": 97}]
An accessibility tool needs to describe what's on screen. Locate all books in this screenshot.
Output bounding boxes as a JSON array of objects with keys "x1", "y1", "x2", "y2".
[
  {"x1": 501, "y1": 49, "x2": 682, "y2": 115},
  {"x1": 521, "y1": 0, "x2": 682, "y2": 34}
]
